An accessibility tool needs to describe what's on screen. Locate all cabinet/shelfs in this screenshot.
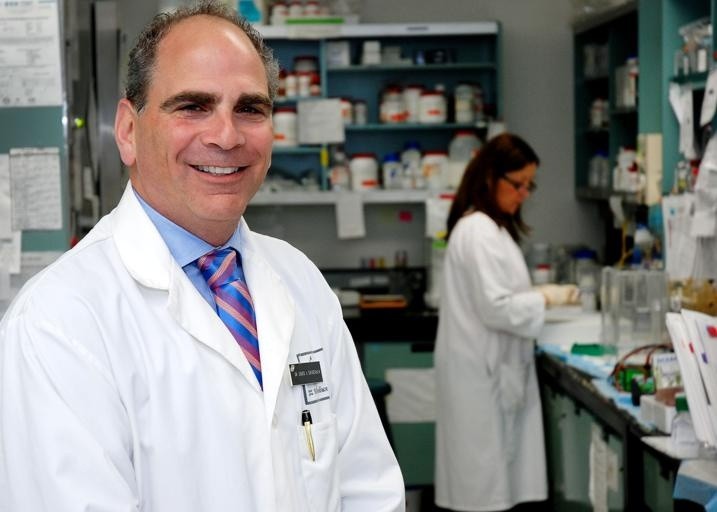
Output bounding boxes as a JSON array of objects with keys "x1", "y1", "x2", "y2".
[
  {"x1": 248, "y1": 19, "x2": 505, "y2": 192},
  {"x1": 571, "y1": 1, "x2": 717, "y2": 209}
]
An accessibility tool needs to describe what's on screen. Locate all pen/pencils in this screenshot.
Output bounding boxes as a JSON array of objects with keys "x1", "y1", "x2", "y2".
[{"x1": 301, "y1": 410, "x2": 316, "y2": 463}]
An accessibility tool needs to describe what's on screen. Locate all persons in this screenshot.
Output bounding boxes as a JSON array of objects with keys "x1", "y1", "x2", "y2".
[
  {"x1": 434, "y1": 132, "x2": 581, "y2": 512},
  {"x1": 0, "y1": 0, "x2": 406, "y2": 512}
]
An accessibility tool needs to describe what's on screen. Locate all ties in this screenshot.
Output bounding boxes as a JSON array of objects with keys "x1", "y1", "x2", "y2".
[{"x1": 194, "y1": 250, "x2": 262, "y2": 389}]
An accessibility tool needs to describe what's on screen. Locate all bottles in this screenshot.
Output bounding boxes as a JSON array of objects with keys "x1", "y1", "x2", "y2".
[
  {"x1": 677, "y1": 158, "x2": 702, "y2": 195},
  {"x1": 378, "y1": 84, "x2": 447, "y2": 123},
  {"x1": 592, "y1": 99, "x2": 610, "y2": 127},
  {"x1": 621, "y1": 58, "x2": 639, "y2": 108},
  {"x1": 383, "y1": 143, "x2": 450, "y2": 190},
  {"x1": 277, "y1": 55, "x2": 320, "y2": 96},
  {"x1": 270, "y1": 0, "x2": 320, "y2": 24},
  {"x1": 588, "y1": 145, "x2": 639, "y2": 193},
  {"x1": 526, "y1": 241, "x2": 560, "y2": 284},
  {"x1": 600, "y1": 267, "x2": 670, "y2": 365},
  {"x1": 673, "y1": 39, "x2": 709, "y2": 79},
  {"x1": 455, "y1": 82, "x2": 484, "y2": 125},
  {"x1": 671, "y1": 395, "x2": 699, "y2": 459},
  {"x1": 272, "y1": 108, "x2": 300, "y2": 147},
  {"x1": 339, "y1": 96, "x2": 368, "y2": 125},
  {"x1": 331, "y1": 146, "x2": 379, "y2": 191}
]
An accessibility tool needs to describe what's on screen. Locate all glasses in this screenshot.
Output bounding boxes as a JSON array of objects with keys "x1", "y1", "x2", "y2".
[{"x1": 503, "y1": 175, "x2": 536, "y2": 192}]
